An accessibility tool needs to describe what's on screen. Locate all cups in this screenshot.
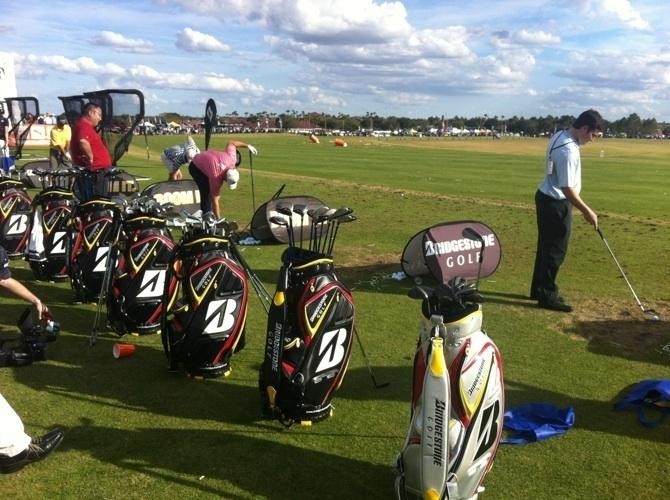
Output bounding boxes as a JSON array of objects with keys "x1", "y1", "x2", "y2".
[{"x1": 112, "y1": 342, "x2": 136, "y2": 359}]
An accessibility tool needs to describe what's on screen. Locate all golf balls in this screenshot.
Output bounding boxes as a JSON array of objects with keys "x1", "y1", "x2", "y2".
[
  {"x1": 653, "y1": 316, "x2": 659, "y2": 322},
  {"x1": 239, "y1": 237, "x2": 261, "y2": 245},
  {"x1": 401, "y1": 194, "x2": 404, "y2": 196},
  {"x1": 391, "y1": 271, "x2": 407, "y2": 281},
  {"x1": 24, "y1": 170, "x2": 35, "y2": 177}
]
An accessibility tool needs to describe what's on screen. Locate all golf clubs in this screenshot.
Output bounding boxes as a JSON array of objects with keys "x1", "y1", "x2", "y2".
[
  {"x1": 407, "y1": 228, "x2": 486, "y2": 321},
  {"x1": 31, "y1": 164, "x2": 81, "y2": 192},
  {"x1": 89, "y1": 208, "x2": 122, "y2": 347},
  {"x1": 1, "y1": 166, "x2": 23, "y2": 182},
  {"x1": 353, "y1": 325, "x2": 390, "y2": 390},
  {"x1": 178, "y1": 210, "x2": 239, "y2": 241},
  {"x1": 270, "y1": 202, "x2": 357, "y2": 259},
  {"x1": 118, "y1": 193, "x2": 179, "y2": 223},
  {"x1": 591, "y1": 218, "x2": 659, "y2": 315},
  {"x1": 79, "y1": 168, "x2": 136, "y2": 200}
]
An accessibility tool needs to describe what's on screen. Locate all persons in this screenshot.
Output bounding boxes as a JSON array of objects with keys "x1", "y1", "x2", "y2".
[
  {"x1": 161, "y1": 133, "x2": 200, "y2": 180},
  {"x1": 49, "y1": 116, "x2": 73, "y2": 170},
  {"x1": 0, "y1": 102, "x2": 10, "y2": 158},
  {"x1": 0, "y1": 239, "x2": 50, "y2": 321},
  {"x1": 20, "y1": 111, "x2": 56, "y2": 124},
  {"x1": 188, "y1": 139, "x2": 258, "y2": 229},
  {"x1": 69, "y1": 103, "x2": 112, "y2": 172},
  {"x1": 527, "y1": 108, "x2": 605, "y2": 315},
  {"x1": 0, "y1": 392, "x2": 66, "y2": 474}
]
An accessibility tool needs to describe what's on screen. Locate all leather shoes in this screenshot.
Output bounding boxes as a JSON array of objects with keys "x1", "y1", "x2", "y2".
[{"x1": 1, "y1": 430, "x2": 63, "y2": 473}]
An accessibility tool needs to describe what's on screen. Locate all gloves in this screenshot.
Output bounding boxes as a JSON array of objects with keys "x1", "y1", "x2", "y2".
[
  {"x1": 64, "y1": 152, "x2": 71, "y2": 160},
  {"x1": 248, "y1": 145, "x2": 258, "y2": 156}
]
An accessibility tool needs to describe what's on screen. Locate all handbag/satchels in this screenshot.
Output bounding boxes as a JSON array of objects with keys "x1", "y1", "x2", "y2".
[
  {"x1": 2, "y1": 155, "x2": 16, "y2": 171},
  {"x1": 614, "y1": 379, "x2": 669, "y2": 428},
  {"x1": 501, "y1": 403, "x2": 576, "y2": 445}
]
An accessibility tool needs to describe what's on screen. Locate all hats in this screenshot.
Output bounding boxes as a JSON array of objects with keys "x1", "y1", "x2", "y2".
[
  {"x1": 57, "y1": 115, "x2": 65, "y2": 124},
  {"x1": 186, "y1": 149, "x2": 196, "y2": 163},
  {"x1": 226, "y1": 169, "x2": 240, "y2": 190}
]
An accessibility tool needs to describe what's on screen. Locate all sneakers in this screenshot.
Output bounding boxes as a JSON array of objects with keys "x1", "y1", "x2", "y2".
[{"x1": 530, "y1": 291, "x2": 572, "y2": 312}]
